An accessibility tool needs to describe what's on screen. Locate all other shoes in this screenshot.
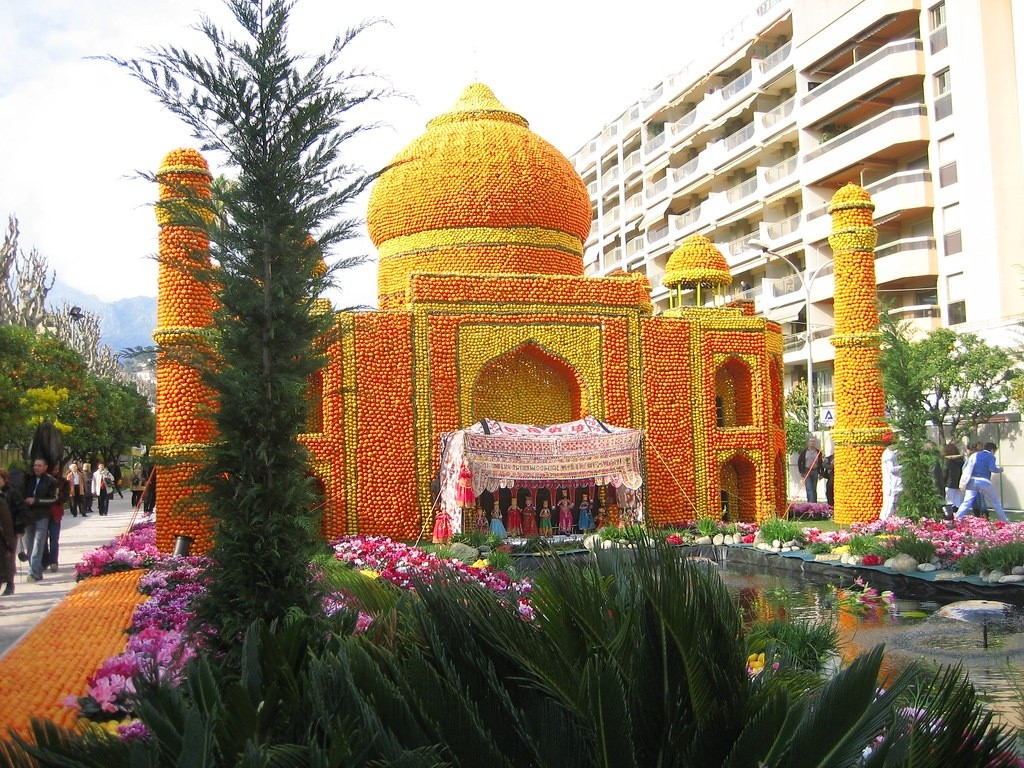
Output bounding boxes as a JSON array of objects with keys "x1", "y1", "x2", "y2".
[
  {"x1": 27, "y1": 576, "x2": 43, "y2": 582},
  {"x1": 50, "y1": 562, "x2": 58, "y2": 572},
  {"x1": 83, "y1": 513, "x2": 87, "y2": 517},
  {"x1": 43, "y1": 558, "x2": 49, "y2": 570},
  {"x1": 74, "y1": 513, "x2": 77, "y2": 517},
  {"x1": 1, "y1": 584, "x2": 15, "y2": 595}
]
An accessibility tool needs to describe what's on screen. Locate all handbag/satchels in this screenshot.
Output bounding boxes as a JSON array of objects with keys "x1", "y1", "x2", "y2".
[
  {"x1": 959, "y1": 452, "x2": 976, "y2": 491},
  {"x1": 106, "y1": 478, "x2": 112, "y2": 487},
  {"x1": 130, "y1": 474, "x2": 140, "y2": 485},
  {"x1": 18, "y1": 538, "x2": 28, "y2": 562}
]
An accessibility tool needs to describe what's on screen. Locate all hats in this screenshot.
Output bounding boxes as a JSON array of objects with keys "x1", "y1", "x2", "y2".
[{"x1": 0, "y1": 468, "x2": 9, "y2": 479}]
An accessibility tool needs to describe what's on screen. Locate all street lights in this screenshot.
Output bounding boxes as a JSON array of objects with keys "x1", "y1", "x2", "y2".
[{"x1": 747, "y1": 236, "x2": 835, "y2": 433}]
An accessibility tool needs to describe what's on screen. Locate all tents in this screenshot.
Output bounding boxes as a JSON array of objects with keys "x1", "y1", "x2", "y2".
[{"x1": 415, "y1": 415, "x2": 711, "y2": 549}]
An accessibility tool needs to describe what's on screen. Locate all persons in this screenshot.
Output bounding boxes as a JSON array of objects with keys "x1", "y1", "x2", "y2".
[
  {"x1": 922, "y1": 441, "x2": 989, "y2": 520},
  {"x1": 953, "y1": 441, "x2": 1010, "y2": 523},
  {"x1": 822, "y1": 453, "x2": 834, "y2": 507},
  {"x1": 740, "y1": 280, "x2": 752, "y2": 299},
  {"x1": 19, "y1": 453, "x2": 157, "y2": 586},
  {"x1": 0, "y1": 466, "x2": 26, "y2": 596},
  {"x1": 798, "y1": 437, "x2": 825, "y2": 504},
  {"x1": 490, "y1": 487, "x2": 606, "y2": 538}
]
